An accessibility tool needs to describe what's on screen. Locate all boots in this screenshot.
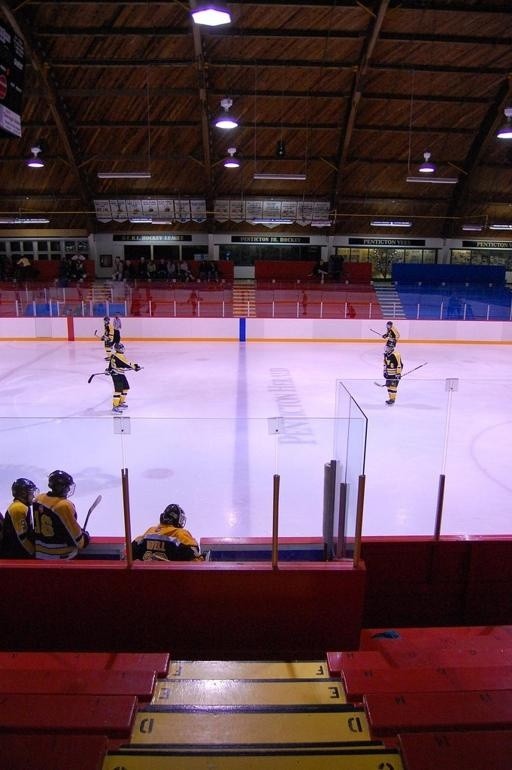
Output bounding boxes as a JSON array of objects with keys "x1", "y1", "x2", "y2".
[
  {"x1": 384, "y1": 398, "x2": 396, "y2": 405},
  {"x1": 111, "y1": 401, "x2": 129, "y2": 415},
  {"x1": 104, "y1": 355, "x2": 111, "y2": 362}
]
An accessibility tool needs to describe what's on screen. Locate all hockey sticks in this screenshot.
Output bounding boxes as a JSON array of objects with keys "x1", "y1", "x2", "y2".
[
  {"x1": 374, "y1": 362, "x2": 428, "y2": 387},
  {"x1": 94, "y1": 330, "x2": 101, "y2": 338},
  {"x1": 83, "y1": 496, "x2": 102, "y2": 531},
  {"x1": 88, "y1": 367, "x2": 143, "y2": 383}
]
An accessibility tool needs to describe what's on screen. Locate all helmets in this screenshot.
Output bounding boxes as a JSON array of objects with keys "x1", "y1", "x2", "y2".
[
  {"x1": 11, "y1": 478, "x2": 39, "y2": 506},
  {"x1": 115, "y1": 343, "x2": 127, "y2": 354},
  {"x1": 102, "y1": 317, "x2": 110, "y2": 322},
  {"x1": 47, "y1": 468, "x2": 75, "y2": 499},
  {"x1": 384, "y1": 339, "x2": 396, "y2": 353},
  {"x1": 159, "y1": 503, "x2": 187, "y2": 529}
]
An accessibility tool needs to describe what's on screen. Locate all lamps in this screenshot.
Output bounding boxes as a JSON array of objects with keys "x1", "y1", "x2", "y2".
[
  {"x1": 223, "y1": 148, "x2": 241, "y2": 167},
  {"x1": 417, "y1": 147, "x2": 436, "y2": 173},
  {"x1": 0, "y1": 218, "x2": 50, "y2": 224},
  {"x1": 369, "y1": 221, "x2": 412, "y2": 227},
  {"x1": 406, "y1": 176, "x2": 458, "y2": 184},
  {"x1": 25, "y1": 146, "x2": 46, "y2": 168},
  {"x1": 212, "y1": 98, "x2": 239, "y2": 130},
  {"x1": 496, "y1": 108, "x2": 512, "y2": 138},
  {"x1": 253, "y1": 173, "x2": 306, "y2": 181},
  {"x1": 460, "y1": 224, "x2": 512, "y2": 231},
  {"x1": 97, "y1": 172, "x2": 152, "y2": 179},
  {"x1": 253, "y1": 218, "x2": 293, "y2": 226},
  {"x1": 190, "y1": 0, "x2": 233, "y2": 26}
]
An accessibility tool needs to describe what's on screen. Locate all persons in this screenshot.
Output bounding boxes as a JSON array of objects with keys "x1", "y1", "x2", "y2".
[
  {"x1": 383, "y1": 345, "x2": 403, "y2": 405},
  {"x1": 313, "y1": 258, "x2": 328, "y2": 283},
  {"x1": 130, "y1": 502, "x2": 206, "y2": 563},
  {"x1": 299, "y1": 289, "x2": 309, "y2": 316},
  {"x1": 131, "y1": 293, "x2": 146, "y2": 318},
  {"x1": 113, "y1": 312, "x2": 121, "y2": 350},
  {"x1": 1, "y1": 476, "x2": 40, "y2": 562},
  {"x1": 188, "y1": 290, "x2": 198, "y2": 314},
  {"x1": 100, "y1": 315, "x2": 116, "y2": 362},
  {"x1": 143, "y1": 295, "x2": 156, "y2": 318},
  {"x1": 32, "y1": 467, "x2": 91, "y2": 562},
  {"x1": 104, "y1": 343, "x2": 142, "y2": 414},
  {"x1": 344, "y1": 300, "x2": 357, "y2": 319},
  {"x1": 0, "y1": 251, "x2": 220, "y2": 289},
  {"x1": 456, "y1": 296, "x2": 475, "y2": 321},
  {"x1": 444, "y1": 289, "x2": 458, "y2": 319},
  {"x1": 381, "y1": 320, "x2": 400, "y2": 361}
]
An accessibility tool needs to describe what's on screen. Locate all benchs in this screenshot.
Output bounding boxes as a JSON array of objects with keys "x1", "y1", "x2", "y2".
[
  {"x1": 327, "y1": 626, "x2": 512, "y2": 770},
  {"x1": 0, "y1": 652, "x2": 172, "y2": 770}
]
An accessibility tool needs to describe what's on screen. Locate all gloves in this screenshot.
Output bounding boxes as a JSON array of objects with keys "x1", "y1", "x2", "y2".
[{"x1": 135, "y1": 364, "x2": 140, "y2": 372}]
